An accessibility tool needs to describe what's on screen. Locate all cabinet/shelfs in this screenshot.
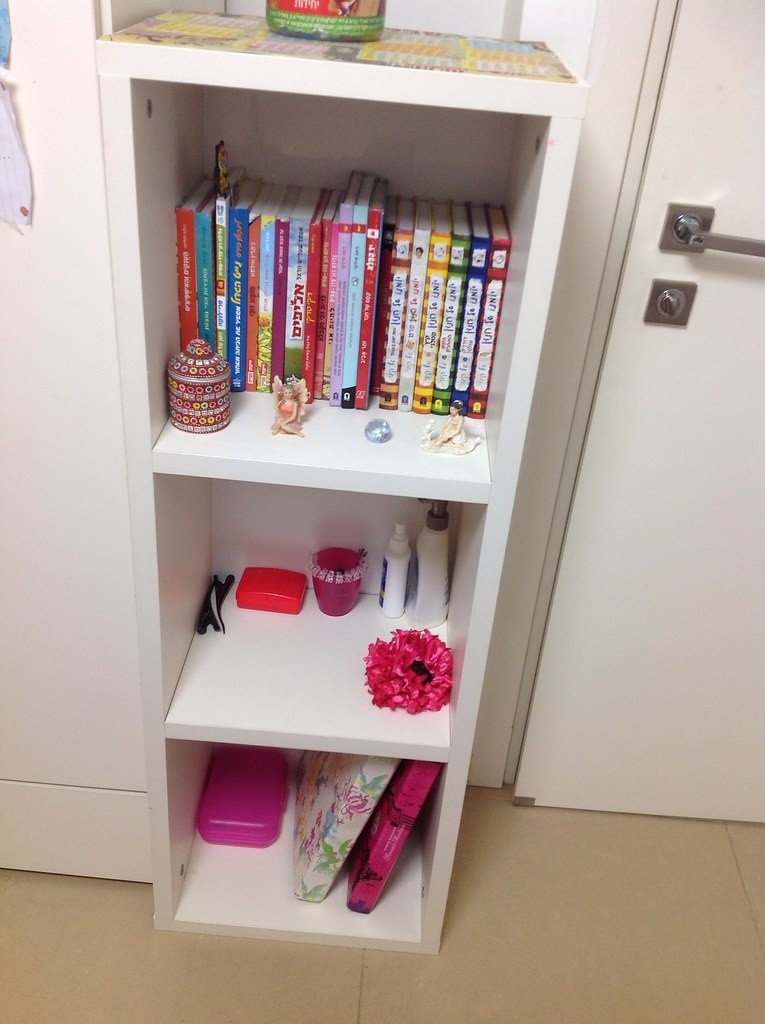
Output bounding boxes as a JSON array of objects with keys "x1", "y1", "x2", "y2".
[
  {"x1": 96, "y1": 6, "x2": 588, "y2": 952},
  {"x1": 0, "y1": 0, "x2": 156, "y2": 884}
]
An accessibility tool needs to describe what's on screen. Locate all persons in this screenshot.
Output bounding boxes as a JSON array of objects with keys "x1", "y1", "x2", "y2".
[
  {"x1": 271, "y1": 384, "x2": 304, "y2": 437},
  {"x1": 328, "y1": 0, "x2": 381, "y2": 17},
  {"x1": 436, "y1": 400, "x2": 467, "y2": 449}
]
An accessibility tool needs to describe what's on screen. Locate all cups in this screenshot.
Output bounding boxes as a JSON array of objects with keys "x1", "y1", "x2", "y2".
[{"x1": 311, "y1": 546, "x2": 365, "y2": 616}]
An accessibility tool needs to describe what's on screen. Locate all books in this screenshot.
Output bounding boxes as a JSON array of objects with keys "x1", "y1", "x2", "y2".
[
  {"x1": 296, "y1": 750, "x2": 446, "y2": 914},
  {"x1": 175, "y1": 140, "x2": 513, "y2": 420}
]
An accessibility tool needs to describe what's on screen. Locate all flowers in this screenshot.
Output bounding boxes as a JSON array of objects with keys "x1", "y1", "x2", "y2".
[{"x1": 363, "y1": 627, "x2": 454, "y2": 715}]
